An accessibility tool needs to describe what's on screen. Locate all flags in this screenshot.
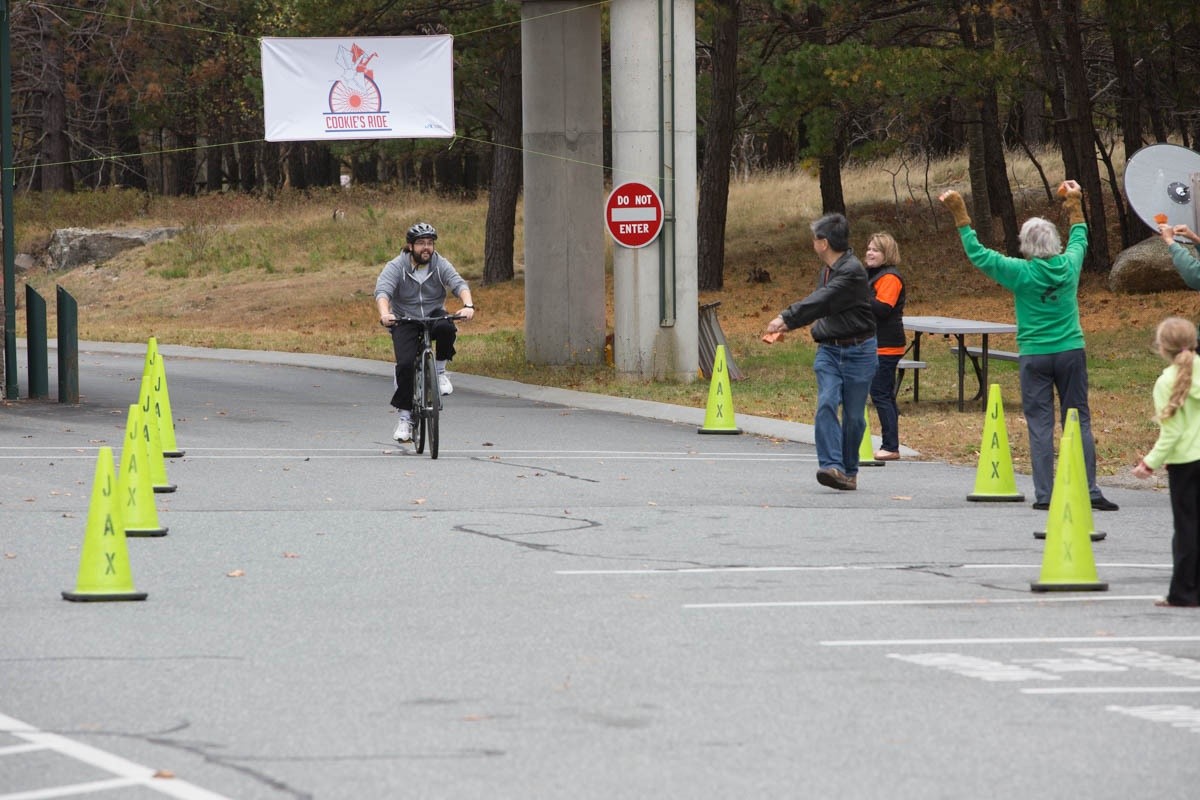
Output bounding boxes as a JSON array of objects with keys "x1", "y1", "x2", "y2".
[{"x1": 262, "y1": 33, "x2": 456, "y2": 142}]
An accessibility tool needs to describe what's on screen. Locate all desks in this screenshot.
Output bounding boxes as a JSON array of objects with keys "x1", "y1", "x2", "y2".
[{"x1": 900, "y1": 315, "x2": 1020, "y2": 412}]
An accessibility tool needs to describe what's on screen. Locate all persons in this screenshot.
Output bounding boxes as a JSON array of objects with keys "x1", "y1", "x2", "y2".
[
  {"x1": 863, "y1": 230, "x2": 907, "y2": 461},
  {"x1": 761, "y1": 211, "x2": 880, "y2": 490},
  {"x1": 373, "y1": 222, "x2": 475, "y2": 443},
  {"x1": 939, "y1": 179, "x2": 1119, "y2": 510},
  {"x1": 1129, "y1": 316, "x2": 1200, "y2": 607},
  {"x1": 1157, "y1": 223, "x2": 1200, "y2": 291}
]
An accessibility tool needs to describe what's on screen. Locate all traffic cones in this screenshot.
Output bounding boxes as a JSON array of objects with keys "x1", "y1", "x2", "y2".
[
  {"x1": 1029, "y1": 439, "x2": 1110, "y2": 593},
  {"x1": 696, "y1": 345, "x2": 744, "y2": 435},
  {"x1": 135, "y1": 334, "x2": 185, "y2": 494},
  {"x1": 117, "y1": 404, "x2": 170, "y2": 537},
  {"x1": 965, "y1": 383, "x2": 1026, "y2": 503},
  {"x1": 1033, "y1": 407, "x2": 1107, "y2": 542},
  {"x1": 61, "y1": 446, "x2": 150, "y2": 602},
  {"x1": 857, "y1": 405, "x2": 886, "y2": 466}
]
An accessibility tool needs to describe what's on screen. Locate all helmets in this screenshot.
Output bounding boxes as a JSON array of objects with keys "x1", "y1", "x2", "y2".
[{"x1": 406, "y1": 222, "x2": 437, "y2": 243}]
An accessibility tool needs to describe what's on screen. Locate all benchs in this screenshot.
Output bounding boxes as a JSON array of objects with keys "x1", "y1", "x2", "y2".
[
  {"x1": 893, "y1": 360, "x2": 927, "y2": 398},
  {"x1": 951, "y1": 346, "x2": 1020, "y2": 401}
]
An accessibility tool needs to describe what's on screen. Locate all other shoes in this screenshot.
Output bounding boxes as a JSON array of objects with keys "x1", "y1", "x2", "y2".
[
  {"x1": 1033, "y1": 502, "x2": 1050, "y2": 510},
  {"x1": 816, "y1": 468, "x2": 848, "y2": 489},
  {"x1": 1155, "y1": 598, "x2": 1171, "y2": 605},
  {"x1": 839, "y1": 477, "x2": 857, "y2": 490},
  {"x1": 874, "y1": 450, "x2": 901, "y2": 461},
  {"x1": 1091, "y1": 496, "x2": 1118, "y2": 510}
]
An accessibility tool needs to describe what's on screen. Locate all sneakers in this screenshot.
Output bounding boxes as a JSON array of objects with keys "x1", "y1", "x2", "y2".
[
  {"x1": 437, "y1": 373, "x2": 453, "y2": 396},
  {"x1": 393, "y1": 417, "x2": 414, "y2": 441}
]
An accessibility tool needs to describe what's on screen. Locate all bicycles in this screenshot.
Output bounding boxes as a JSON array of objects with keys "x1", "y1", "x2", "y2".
[{"x1": 381, "y1": 311, "x2": 474, "y2": 459}]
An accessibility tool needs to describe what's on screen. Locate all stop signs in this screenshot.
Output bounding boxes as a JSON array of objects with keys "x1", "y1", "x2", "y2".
[{"x1": 604, "y1": 183, "x2": 665, "y2": 249}]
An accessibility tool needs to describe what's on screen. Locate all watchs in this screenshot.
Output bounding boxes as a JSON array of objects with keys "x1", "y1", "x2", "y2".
[{"x1": 463, "y1": 303, "x2": 475, "y2": 310}]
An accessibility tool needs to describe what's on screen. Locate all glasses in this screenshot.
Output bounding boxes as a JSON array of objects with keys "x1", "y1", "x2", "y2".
[{"x1": 412, "y1": 240, "x2": 434, "y2": 248}]
